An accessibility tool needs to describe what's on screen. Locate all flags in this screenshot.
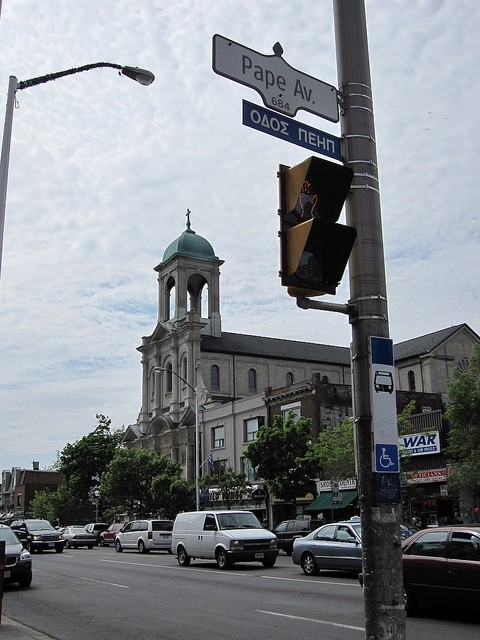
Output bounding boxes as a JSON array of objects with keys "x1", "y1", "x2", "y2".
[{"x1": 207, "y1": 455, "x2": 215, "y2": 472}]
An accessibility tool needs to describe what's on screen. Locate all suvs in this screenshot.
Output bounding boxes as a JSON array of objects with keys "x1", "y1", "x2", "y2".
[{"x1": 9, "y1": 519, "x2": 64, "y2": 554}]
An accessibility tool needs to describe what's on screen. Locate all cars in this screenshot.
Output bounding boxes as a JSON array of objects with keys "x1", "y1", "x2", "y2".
[
  {"x1": 114, "y1": 519, "x2": 174, "y2": 553},
  {"x1": 58, "y1": 527, "x2": 98, "y2": 549},
  {"x1": 85, "y1": 523, "x2": 109, "y2": 546},
  {"x1": 271, "y1": 519, "x2": 325, "y2": 555},
  {"x1": 99, "y1": 523, "x2": 125, "y2": 547},
  {"x1": 0, "y1": 523, "x2": 32, "y2": 588},
  {"x1": 291, "y1": 519, "x2": 418, "y2": 576},
  {"x1": 358, "y1": 527, "x2": 480, "y2": 617}
]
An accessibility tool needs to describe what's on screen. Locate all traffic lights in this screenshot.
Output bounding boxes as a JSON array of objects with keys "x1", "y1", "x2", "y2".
[{"x1": 287, "y1": 155, "x2": 357, "y2": 294}]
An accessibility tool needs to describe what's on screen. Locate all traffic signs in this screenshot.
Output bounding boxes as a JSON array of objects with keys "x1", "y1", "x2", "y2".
[
  {"x1": 214, "y1": 36, "x2": 337, "y2": 123},
  {"x1": 241, "y1": 99, "x2": 339, "y2": 160}
]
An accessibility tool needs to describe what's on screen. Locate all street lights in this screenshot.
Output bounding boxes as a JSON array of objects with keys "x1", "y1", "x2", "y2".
[
  {"x1": 153, "y1": 367, "x2": 200, "y2": 510},
  {"x1": 1, "y1": 61, "x2": 155, "y2": 268}
]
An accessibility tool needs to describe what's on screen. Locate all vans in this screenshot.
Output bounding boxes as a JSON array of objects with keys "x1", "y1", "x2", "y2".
[{"x1": 170, "y1": 510, "x2": 279, "y2": 569}]
